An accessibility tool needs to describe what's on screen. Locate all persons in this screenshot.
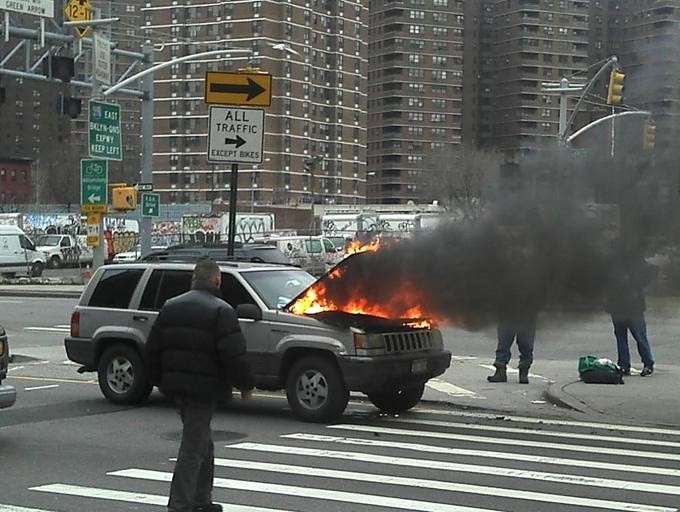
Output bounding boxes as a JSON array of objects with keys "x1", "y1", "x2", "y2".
[
  {"x1": 143, "y1": 260, "x2": 254, "y2": 512},
  {"x1": 603, "y1": 252, "x2": 654, "y2": 377},
  {"x1": 487, "y1": 309, "x2": 536, "y2": 384}
]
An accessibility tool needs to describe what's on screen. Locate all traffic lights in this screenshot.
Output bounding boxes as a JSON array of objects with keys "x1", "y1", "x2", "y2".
[
  {"x1": 641, "y1": 124, "x2": 656, "y2": 152},
  {"x1": 606, "y1": 71, "x2": 626, "y2": 106}
]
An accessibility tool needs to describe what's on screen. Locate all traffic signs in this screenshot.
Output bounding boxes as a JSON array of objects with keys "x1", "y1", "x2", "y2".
[
  {"x1": 82, "y1": 182, "x2": 106, "y2": 204},
  {"x1": 143, "y1": 194, "x2": 160, "y2": 215},
  {"x1": 204, "y1": 70, "x2": 273, "y2": 109},
  {"x1": 207, "y1": 105, "x2": 264, "y2": 165},
  {"x1": 133, "y1": 182, "x2": 154, "y2": 191},
  {"x1": 1, "y1": 2, "x2": 58, "y2": 18}
]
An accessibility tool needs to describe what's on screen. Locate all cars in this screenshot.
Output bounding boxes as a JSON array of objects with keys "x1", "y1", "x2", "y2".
[
  {"x1": 265, "y1": 229, "x2": 297, "y2": 236},
  {"x1": 112, "y1": 245, "x2": 167, "y2": 264}
]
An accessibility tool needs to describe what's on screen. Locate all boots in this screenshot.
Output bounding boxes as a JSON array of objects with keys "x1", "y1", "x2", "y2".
[
  {"x1": 488, "y1": 366, "x2": 507, "y2": 384},
  {"x1": 518, "y1": 367, "x2": 529, "y2": 383}
]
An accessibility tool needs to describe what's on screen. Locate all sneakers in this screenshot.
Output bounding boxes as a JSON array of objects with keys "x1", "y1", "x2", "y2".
[
  {"x1": 194, "y1": 504, "x2": 222, "y2": 512},
  {"x1": 640, "y1": 364, "x2": 653, "y2": 376},
  {"x1": 619, "y1": 366, "x2": 630, "y2": 375}
]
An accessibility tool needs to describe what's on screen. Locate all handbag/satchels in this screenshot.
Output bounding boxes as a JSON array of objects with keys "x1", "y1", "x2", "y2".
[{"x1": 577, "y1": 355, "x2": 623, "y2": 384}]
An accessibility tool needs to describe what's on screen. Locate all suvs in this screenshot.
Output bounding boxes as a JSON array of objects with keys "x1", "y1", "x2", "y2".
[
  {"x1": 145, "y1": 244, "x2": 291, "y2": 265},
  {"x1": 66, "y1": 250, "x2": 453, "y2": 422},
  {"x1": 0, "y1": 327, "x2": 16, "y2": 410}
]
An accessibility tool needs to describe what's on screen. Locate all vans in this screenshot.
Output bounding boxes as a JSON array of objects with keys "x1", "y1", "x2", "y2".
[
  {"x1": 1, "y1": 226, "x2": 46, "y2": 277},
  {"x1": 254, "y1": 237, "x2": 343, "y2": 274}
]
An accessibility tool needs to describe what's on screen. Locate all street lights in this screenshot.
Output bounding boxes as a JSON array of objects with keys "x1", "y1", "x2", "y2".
[{"x1": 353, "y1": 172, "x2": 375, "y2": 204}]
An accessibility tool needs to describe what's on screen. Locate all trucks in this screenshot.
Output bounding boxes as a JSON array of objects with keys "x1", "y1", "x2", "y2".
[
  {"x1": 321, "y1": 213, "x2": 441, "y2": 240},
  {"x1": 36, "y1": 234, "x2": 109, "y2": 268}
]
